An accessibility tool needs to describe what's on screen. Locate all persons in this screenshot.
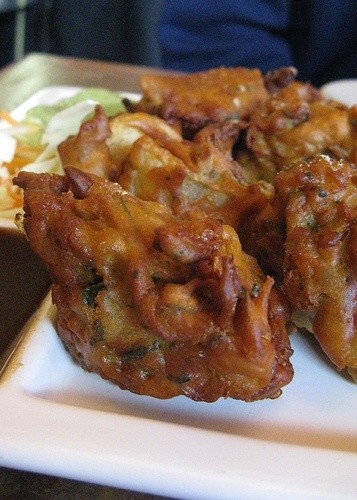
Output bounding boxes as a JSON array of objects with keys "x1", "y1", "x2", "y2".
[{"x1": 156, "y1": 0, "x2": 357, "y2": 88}]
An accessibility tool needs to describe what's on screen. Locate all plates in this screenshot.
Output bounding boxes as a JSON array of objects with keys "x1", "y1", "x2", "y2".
[
  {"x1": 0, "y1": 285, "x2": 356, "y2": 499},
  {"x1": 0, "y1": 51, "x2": 192, "y2": 238}
]
